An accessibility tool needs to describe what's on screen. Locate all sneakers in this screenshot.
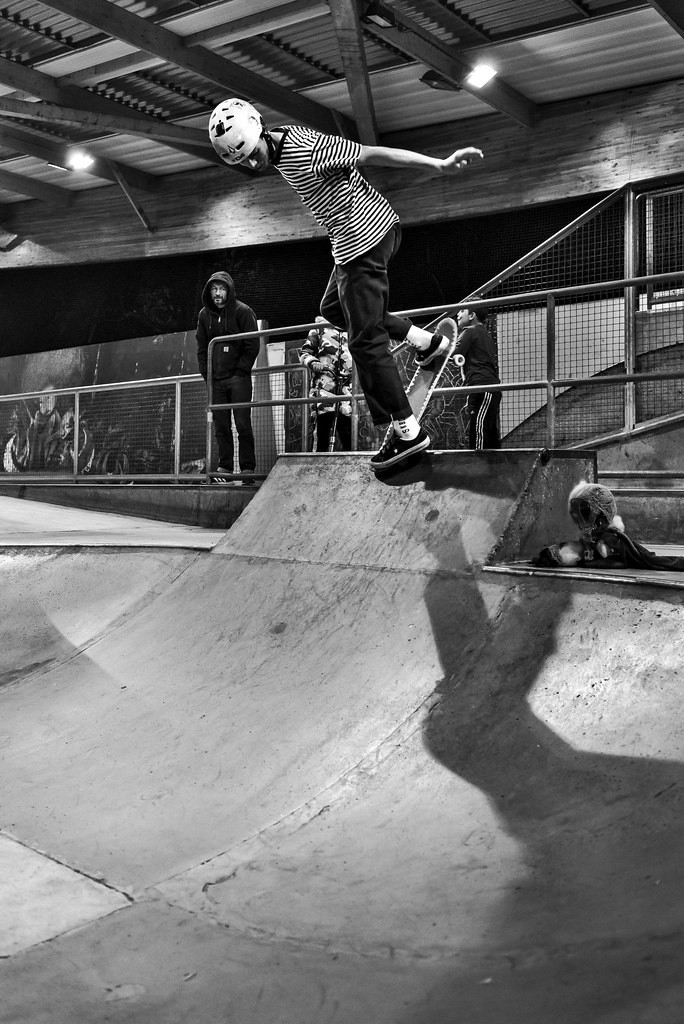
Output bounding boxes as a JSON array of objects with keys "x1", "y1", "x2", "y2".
[
  {"x1": 241, "y1": 480, "x2": 255, "y2": 485},
  {"x1": 369, "y1": 427, "x2": 430, "y2": 468},
  {"x1": 414, "y1": 334, "x2": 449, "y2": 366},
  {"x1": 201, "y1": 470, "x2": 234, "y2": 485}
]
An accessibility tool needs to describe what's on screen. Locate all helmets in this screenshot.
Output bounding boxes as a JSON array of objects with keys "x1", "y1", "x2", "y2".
[{"x1": 209, "y1": 98, "x2": 263, "y2": 164}]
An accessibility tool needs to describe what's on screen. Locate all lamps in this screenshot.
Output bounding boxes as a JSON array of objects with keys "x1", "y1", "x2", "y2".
[{"x1": 420, "y1": 69, "x2": 460, "y2": 92}]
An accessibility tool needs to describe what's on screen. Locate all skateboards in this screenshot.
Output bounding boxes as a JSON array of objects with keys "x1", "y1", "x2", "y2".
[{"x1": 377, "y1": 317, "x2": 466, "y2": 453}]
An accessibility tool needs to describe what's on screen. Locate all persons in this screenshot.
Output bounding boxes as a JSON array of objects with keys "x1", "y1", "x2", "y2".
[
  {"x1": 209, "y1": 99, "x2": 484, "y2": 468},
  {"x1": 448, "y1": 296, "x2": 502, "y2": 449},
  {"x1": 299, "y1": 316, "x2": 354, "y2": 452},
  {"x1": 196, "y1": 272, "x2": 260, "y2": 485}
]
